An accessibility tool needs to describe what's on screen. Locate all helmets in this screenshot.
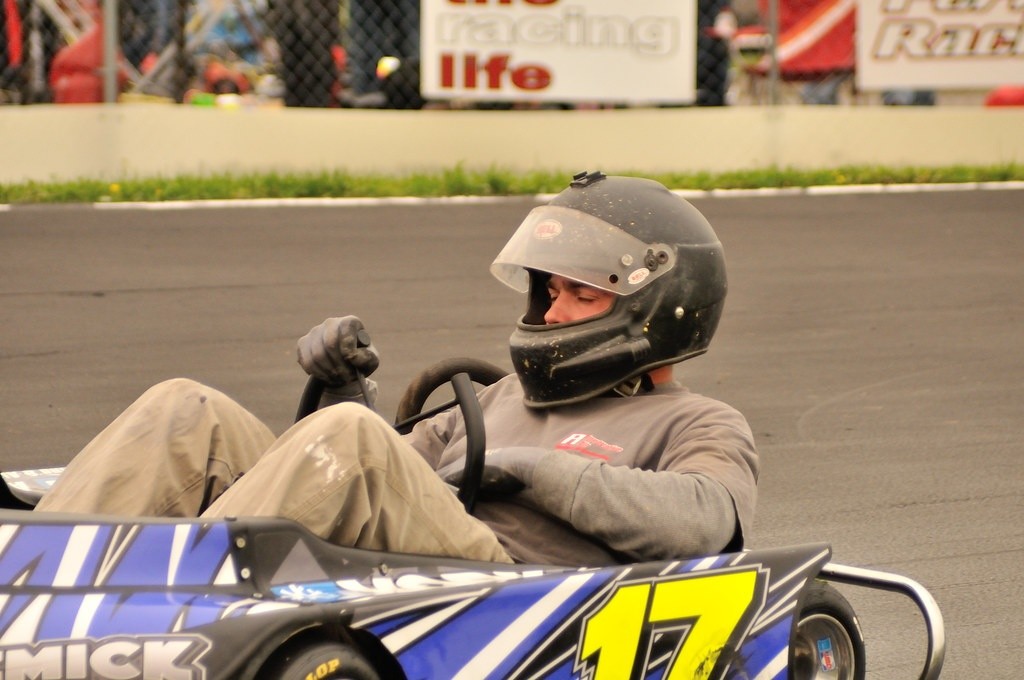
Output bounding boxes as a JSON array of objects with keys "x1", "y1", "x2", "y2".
[{"x1": 488, "y1": 169, "x2": 730, "y2": 410}]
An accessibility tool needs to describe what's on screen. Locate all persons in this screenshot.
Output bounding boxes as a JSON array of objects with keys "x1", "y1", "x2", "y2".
[
  {"x1": 271, "y1": 0, "x2": 425, "y2": 109},
  {"x1": 660, "y1": 0, "x2": 734, "y2": 109},
  {"x1": 34, "y1": 172, "x2": 760, "y2": 568}
]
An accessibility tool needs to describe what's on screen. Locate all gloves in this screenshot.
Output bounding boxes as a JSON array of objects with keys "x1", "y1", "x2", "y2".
[
  {"x1": 293, "y1": 314, "x2": 382, "y2": 398},
  {"x1": 430, "y1": 443, "x2": 551, "y2": 501}
]
